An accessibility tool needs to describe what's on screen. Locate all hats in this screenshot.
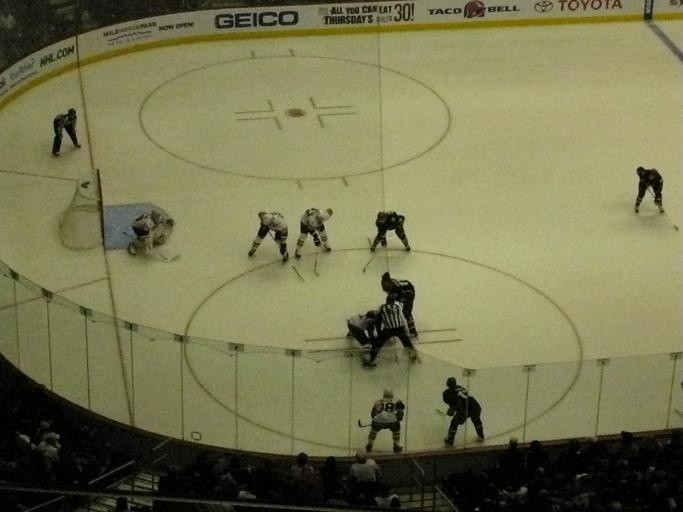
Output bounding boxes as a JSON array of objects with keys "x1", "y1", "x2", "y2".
[{"x1": 47, "y1": 432, "x2": 60, "y2": 440}]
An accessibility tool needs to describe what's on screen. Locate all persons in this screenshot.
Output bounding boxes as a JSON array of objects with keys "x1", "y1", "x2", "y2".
[
  {"x1": 442, "y1": 376, "x2": 485, "y2": 446},
  {"x1": 635, "y1": 167, "x2": 663, "y2": 214},
  {"x1": 453, "y1": 437, "x2": 683, "y2": 512},
  {"x1": 248, "y1": 212, "x2": 290, "y2": 263},
  {"x1": 52, "y1": 109, "x2": 81, "y2": 157},
  {"x1": 0, "y1": 419, "x2": 112, "y2": 490},
  {"x1": 158, "y1": 448, "x2": 406, "y2": 512},
  {"x1": 130, "y1": 210, "x2": 160, "y2": 255},
  {"x1": 1, "y1": 0, "x2": 204, "y2": 74},
  {"x1": 295, "y1": 207, "x2": 333, "y2": 259},
  {"x1": 365, "y1": 388, "x2": 404, "y2": 454},
  {"x1": 371, "y1": 211, "x2": 411, "y2": 254},
  {"x1": 344, "y1": 273, "x2": 417, "y2": 367}
]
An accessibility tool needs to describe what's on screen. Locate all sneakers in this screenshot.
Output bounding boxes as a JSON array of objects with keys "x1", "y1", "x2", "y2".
[
  {"x1": 366, "y1": 444, "x2": 372, "y2": 449},
  {"x1": 478, "y1": 432, "x2": 485, "y2": 439},
  {"x1": 52, "y1": 149, "x2": 60, "y2": 155},
  {"x1": 295, "y1": 251, "x2": 301, "y2": 257},
  {"x1": 635, "y1": 206, "x2": 639, "y2": 212},
  {"x1": 129, "y1": 243, "x2": 136, "y2": 255},
  {"x1": 407, "y1": 247, "x2": 410, "y2": 250},
  {"x1": 323, "y1": 244, "x2": 331, "y2": 250},
  {"x1": 444, "y1": 437, "x2": 453, "y2": 445},
  {"x1": 283, "y1": 252, "x2": 288, "y2": 261},
  {"x1": 74, "y1": 144, "x2": 81, "y2": 147},
  {"x1": 394, "y1": 446, "x2": 403, "y2": 452},
  {"x1": 370, "y1": 247, "x2": 375, "y2": 251},
  {"x1": 660, "y1": 207, "x2": 664, "y2": 212},
  {"x1": 249, "y1": 249, "x2": 256, "y2": 256}
]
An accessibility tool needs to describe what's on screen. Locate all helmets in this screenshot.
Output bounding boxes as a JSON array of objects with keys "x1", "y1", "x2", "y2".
[
  {"x1": 68, "y1": 108, "x2": 76, "y2": 119},
  {"x1": 447, "y1": 377, "x2": 456, "y2": 387},
  {"x1": 262, "y1": 214, "x2": 273, "y2": 226},
  {"x1": 357, "y1": 451, "x2": 368, "y2": 461},
  {"x1": 151, "y1": 209, "x2": 161, "y2": 222},
  {"x1": 383, "y1": 388, "x2": 393, "y2": 399},
  {"x1": 386, "y1": 295, "x2": 395, "y2": 304}
]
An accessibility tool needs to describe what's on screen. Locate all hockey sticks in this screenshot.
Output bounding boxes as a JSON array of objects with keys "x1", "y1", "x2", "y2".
[
  {"x1": 357, "y1": 419, "x2": 372, "y2": 428},
  {"x1": 363, "y1": 232, "x2": 394, "y2": 273},
  {"x1": 434, "y1": 407, "x2": 455, "y2": 416},
  {"x1": 314, "y1": 230, "x2": 320, "y2": 277},
  {"x1": 122, "y1": 230, "x2": 181, "y2": 261},
  {"x1": 648, "y1": 186, "x2": 679, "y2": 230},
  {"x1": 267, "y1": 231, "x2": 306, "y2": 283}
]
